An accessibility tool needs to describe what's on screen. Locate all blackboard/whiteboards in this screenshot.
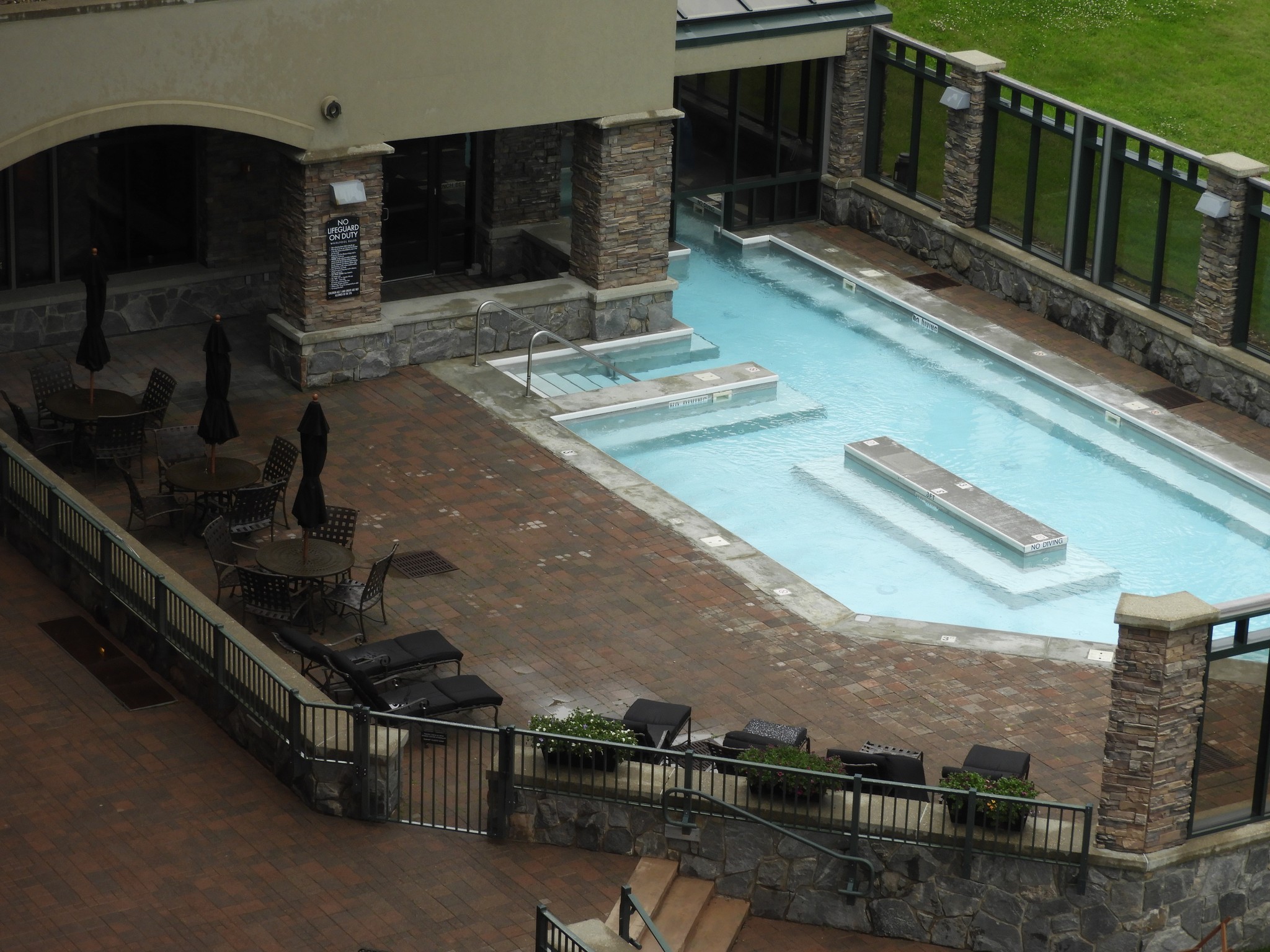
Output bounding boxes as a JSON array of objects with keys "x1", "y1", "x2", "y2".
[{"x1": 325, "y1": 217, "x2": 362, "y2": 300}]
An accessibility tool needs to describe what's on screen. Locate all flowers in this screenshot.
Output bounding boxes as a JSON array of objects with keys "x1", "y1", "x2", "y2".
[
  {"x1": 526, "y1": 707, "x2": 638, "y2": 765},
  {"x1": 939, "y1": 770, "x2": 1040, "y2": 824},
  {"x1": 732, "y1": 743, "x2": 849, "y2": 798}
]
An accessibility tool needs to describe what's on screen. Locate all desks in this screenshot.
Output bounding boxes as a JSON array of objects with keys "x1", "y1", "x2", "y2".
[
  {"x1": 166, "y1": 457, "x2": 261, "y2": 533},
  {"x1": 45, "y1": 388, "x2": 136, "y2": 425},
  {"x1": 666, "y1": 738, "x2": 720, "y2": 771},
  {"x1": 256, "y1": 537, "x2": 355, "y2": 626}
]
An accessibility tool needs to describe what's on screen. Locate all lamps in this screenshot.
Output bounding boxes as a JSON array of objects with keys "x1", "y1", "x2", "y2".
[
  {"x1": 939, "y1": 86, "x2": 971, "y2": 110},
  {"x1": 1194, "y1": 191, "x2": 1231, "y2": 218},
  {"x1": 330, "y1": 180, "x2": 367, "y2": 206}
]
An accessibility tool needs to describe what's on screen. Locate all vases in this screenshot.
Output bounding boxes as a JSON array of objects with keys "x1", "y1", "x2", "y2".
[
  {"x1": 942, "y1": 796, "x2": 1029, "y2": 832},
  {"x1": 749, "y1": 780, "x2": 827, "y2": 803},
  {"x1": 541, "y1": 745, "x2": 617, "y2": 773}
]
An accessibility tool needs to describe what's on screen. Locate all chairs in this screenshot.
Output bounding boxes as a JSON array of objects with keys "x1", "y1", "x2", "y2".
[
  {"x1": 113, "y1": 425, "x2": 301, "y2": 547},
  {"x1": 272, "y1": 628, "x2": 503, "y2": 747},
  {"x1": 702, "y1": 718, "x2": 811, "y2": 776},
  {"x1": 599, "y1": 698, "x2": 692, "y2": 766},
  {"x1": 0, "y1": 359, "x2": 178, "y2": 483},
  {"x1": 202, "y1": 505, "x2": 399, "y2": 641},
  {"x1": 827, "y1": 740, "x2": 931, "y2": 802},
  {"x1": 938, "y1": 745, "x2": 1034, "y2": 804}
]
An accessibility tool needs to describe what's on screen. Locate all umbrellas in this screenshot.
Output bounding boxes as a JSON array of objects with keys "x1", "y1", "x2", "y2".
[
  {"x1": 291, "y1": 394, "x2": 331, "y2": 560},
  {"x1": 76, "y1": 247, "x2": 112, "y2": 405},
  {"x1": 197, "y1": 315, "x2": 239, "y2": 474}
]
